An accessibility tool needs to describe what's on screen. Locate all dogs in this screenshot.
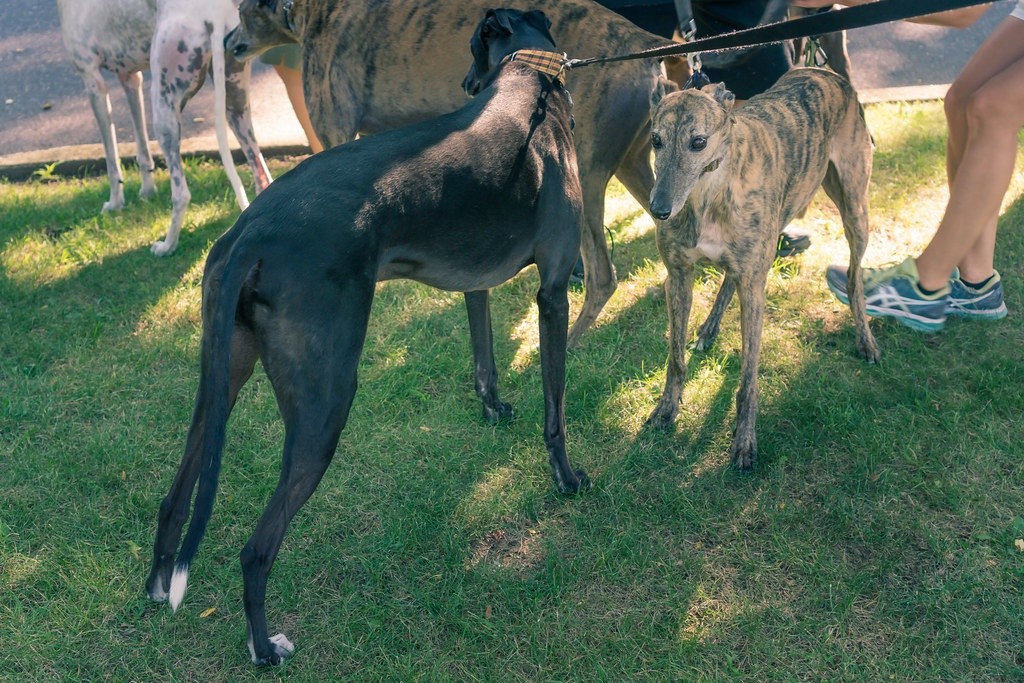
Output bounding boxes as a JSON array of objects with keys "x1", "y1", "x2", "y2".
[
  {"x1": 221, "y1": 0, "x2": 696, "y2": 353},
  {"x1": 144, "y1": 5, "x2": 592, "y2": 671},
  {"x1": 637, "y1": 62, "x2": 884, "y2": 473},
  {"x1": 55, "y1": 0, "x2": 274, "y2": 258}
]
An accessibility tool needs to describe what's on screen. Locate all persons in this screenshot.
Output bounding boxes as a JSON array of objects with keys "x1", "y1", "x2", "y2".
[{"x1": 795, "y1": 0, "x2": 1024, "y2": 333}]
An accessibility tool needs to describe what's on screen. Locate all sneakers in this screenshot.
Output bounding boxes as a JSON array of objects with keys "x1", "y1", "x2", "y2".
[
  {"x1": 942, "y1": 266, "x2": 1007, "y2": 320},
  {"x1": 826, "y1": 255, "x2": 952, "y2": 334},
  {"x1": 775, "y1": 225, "x2": 811, "y2": 257}
]
